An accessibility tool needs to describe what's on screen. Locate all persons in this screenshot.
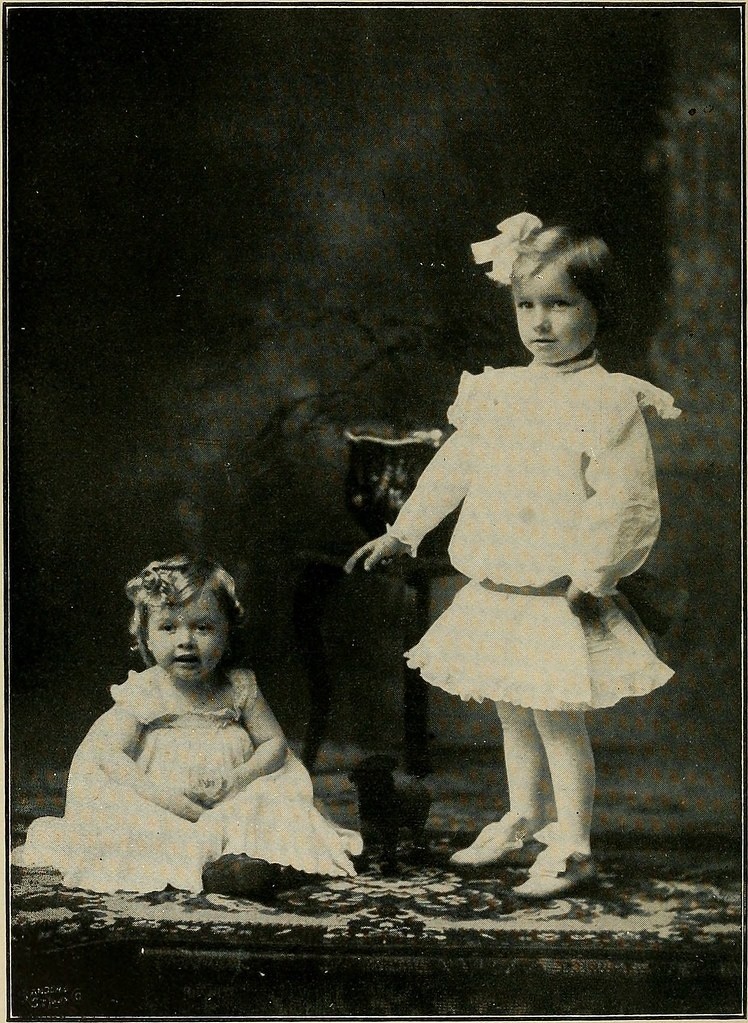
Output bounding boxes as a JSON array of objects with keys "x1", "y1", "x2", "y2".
[
  {"x1": 343, "y1": 207, "x2": 682, "y2": 899},
  {"x1": 8, "y1": 548, "x2": 366, "y2": 897}
]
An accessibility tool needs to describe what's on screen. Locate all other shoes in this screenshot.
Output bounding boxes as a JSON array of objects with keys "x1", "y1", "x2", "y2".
[
  {"x1": 512, "y1": 822, "x2": 597, "y2": 901},
  {"x1": 449, "y1": 811, "x2": 545, "y2": 870}
]
